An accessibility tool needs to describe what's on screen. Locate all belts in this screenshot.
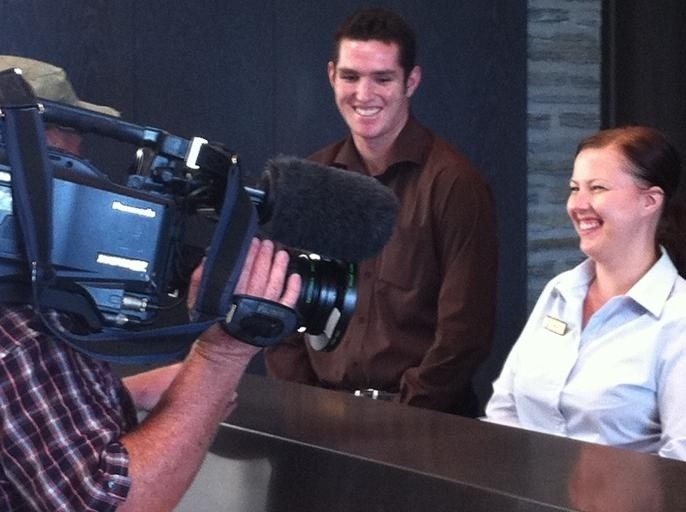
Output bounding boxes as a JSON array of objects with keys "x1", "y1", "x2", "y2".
[{"x1": 340, "y1": 389, "x2": 396, "y2": 402}]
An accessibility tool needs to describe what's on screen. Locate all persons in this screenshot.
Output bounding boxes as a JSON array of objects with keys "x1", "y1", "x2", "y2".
[
  {"x1": 472, "y1": 126, "x2": 686, "y2": 462},
  {"x1": 1, "y1": 53, "x2": 301, "y2": 512},
  {"x1": 262, "y1": 8, "x2": 500, "y2": 421}
]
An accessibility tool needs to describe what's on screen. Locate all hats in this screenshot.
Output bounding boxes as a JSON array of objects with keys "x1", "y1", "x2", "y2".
[{"x1": 0, "y1": 55, "x2": 122, "y2": 129}]
